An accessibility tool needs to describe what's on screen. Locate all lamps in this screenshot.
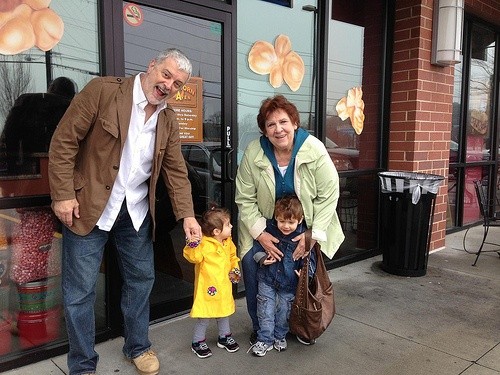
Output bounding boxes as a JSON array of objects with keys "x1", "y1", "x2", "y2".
[{"x1": 430, "y1": 0, "x2": 465, "y2": 67}]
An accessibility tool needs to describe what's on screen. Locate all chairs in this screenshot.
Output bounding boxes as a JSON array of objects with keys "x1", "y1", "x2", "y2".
[{"x1": 471, "y1": 179, "x2": 500, "y2": 266}]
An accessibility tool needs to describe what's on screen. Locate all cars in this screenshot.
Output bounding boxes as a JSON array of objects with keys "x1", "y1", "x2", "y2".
[
  {"x1": 180, "y1": 140, "x2": 224, "y2": 220},
  {"x1": 305, "y1": 129, "x2": 360, "y2": 201},
  {"x1": 449, "y1": 139, "x2": 489, "y2": 175}
]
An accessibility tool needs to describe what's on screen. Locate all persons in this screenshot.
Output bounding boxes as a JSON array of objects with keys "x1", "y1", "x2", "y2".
[
  {"x1": 183, "y1": 200, "x2": 241, "y2": 358},
  {"x1": 0, "y1": 76, "x2": 76, "y2": 176},
  {"x1": 234, "y1": 95, "x2": 345, "y2": 344},
  {"x1": 48, "y1": 51, "x2": 202, "y2": 375},
  {"x1": 253, "y1": 195, "x2": 317, "y2": 356}
]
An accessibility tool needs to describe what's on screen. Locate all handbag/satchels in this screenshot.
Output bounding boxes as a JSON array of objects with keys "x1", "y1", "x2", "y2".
[{"x1": 290, "y1": 232, "x2": 335, "y2": 340}]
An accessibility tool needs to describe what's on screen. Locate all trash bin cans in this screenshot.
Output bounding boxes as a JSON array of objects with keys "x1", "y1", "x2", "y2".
[{"x1": 376, "y1": 171, "x2": 445, "y2": 276}]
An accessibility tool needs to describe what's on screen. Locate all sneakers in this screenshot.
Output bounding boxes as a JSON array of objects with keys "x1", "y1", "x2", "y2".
[
  {"x1": 274, "y1": 338, "x2": 287, "y2": 352},
  {"x1": 296, "y1": 334, "x2": 316, "y2": 345},
  {"x1": 249, "y1": 330, "x2": 259, "y2": 345},
  {"x1": 246, "y1": 340, "x2": 273, "y2": 357},
  {"x1": 191, "y1": 338, "x2": 213, "y2": 359},
  {"x1": 132, "y1": 349, "x2": 159, "y2": 375},
  {"x1": 217, "y1": 333, "x2": 239, "y2": 352}
]
